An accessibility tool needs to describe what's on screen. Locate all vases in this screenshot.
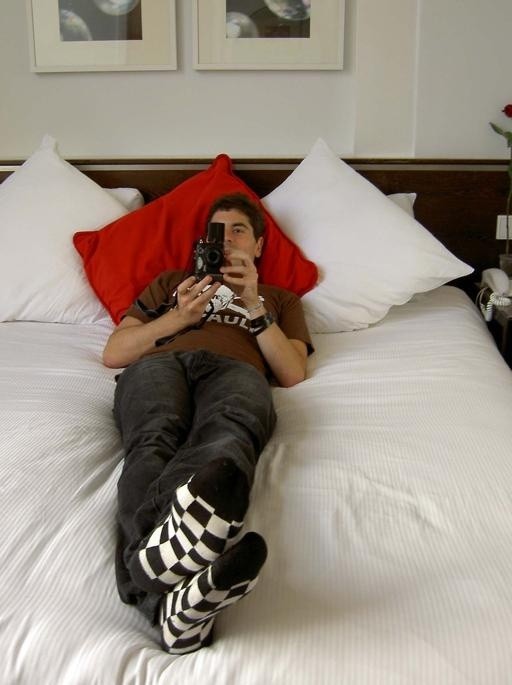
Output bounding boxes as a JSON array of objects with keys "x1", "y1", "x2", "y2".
[{"x1": 500, "y1": 255, "x2": 512, "y2": 280}]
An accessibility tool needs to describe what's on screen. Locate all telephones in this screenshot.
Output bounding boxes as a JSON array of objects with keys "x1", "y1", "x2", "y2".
[{"x1": 482, "y1": 268, "x2": 512, "y2": 298}]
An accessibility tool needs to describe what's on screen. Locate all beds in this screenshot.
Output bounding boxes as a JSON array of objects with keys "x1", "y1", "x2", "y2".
[{"x1": 0, "y1": 284, "x2": 512, "y2": 685}]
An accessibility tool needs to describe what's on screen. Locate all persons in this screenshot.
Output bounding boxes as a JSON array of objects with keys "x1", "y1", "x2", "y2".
[{"x1": 103, "y1": 192, "x2": 315, "y2": 653}]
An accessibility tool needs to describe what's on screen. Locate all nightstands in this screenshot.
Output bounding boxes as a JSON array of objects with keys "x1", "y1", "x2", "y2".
[{"x1": 475, "y1": 282, "x2": 512, "y2": 356}]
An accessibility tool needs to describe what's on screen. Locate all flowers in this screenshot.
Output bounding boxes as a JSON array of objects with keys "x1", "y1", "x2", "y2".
[{"x1": 489, "y1": 104, "x2": 512, "y2": 257}]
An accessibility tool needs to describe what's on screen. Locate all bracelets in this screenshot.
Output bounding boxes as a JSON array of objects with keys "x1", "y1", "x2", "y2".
[
  {"x1": 247, "y1": 303, "x2": 263, "y2": 314},
  {"x1": 250, "y1": 311, "x2": 272, "y2": 337}
]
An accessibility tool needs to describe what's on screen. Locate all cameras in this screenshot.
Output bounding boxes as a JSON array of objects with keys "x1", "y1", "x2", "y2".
[{"x1": 193, "y1": 222, "x2": 225, "y2": 286}]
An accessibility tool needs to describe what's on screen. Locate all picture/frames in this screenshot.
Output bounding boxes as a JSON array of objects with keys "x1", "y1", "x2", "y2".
[
  {"x1": 192, "y1": 0, "x2": 346, "y2": 70},
  {"x1": 25, "y1": 0, "x2": 178, "y2": 72}
]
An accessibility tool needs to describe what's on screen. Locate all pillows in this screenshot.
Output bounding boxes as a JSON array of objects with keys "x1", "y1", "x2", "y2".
[
  {"x1": 0, "y1": 134, "x2": 127, "y2": 327},
  {"x1": 386, "y1": 193, "x2": 417, "y2": 219},
  {"x1": 105, "y1": 188, "x2": 144, "y2": 212},
  {"x1": 261, "y1": 138, "x2": 474, "y2": 334},
  {"x1": 74, "y1": 154, "x2": 316, "y2": 326}
]
